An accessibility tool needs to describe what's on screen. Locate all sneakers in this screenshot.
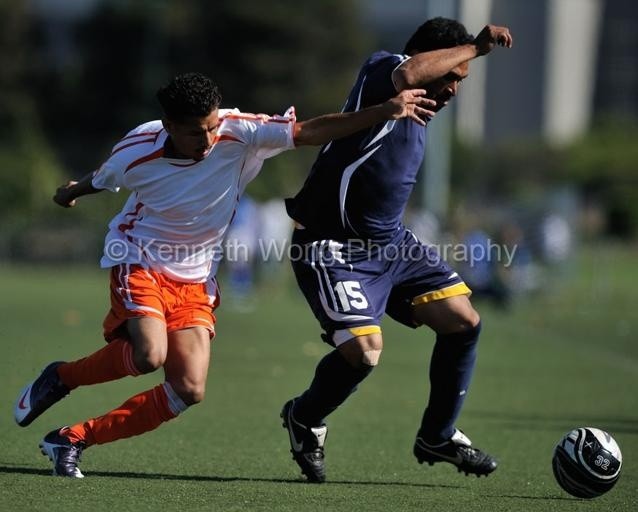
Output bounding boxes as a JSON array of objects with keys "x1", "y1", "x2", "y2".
[
  {"x1": 279, "y1": 397, "x2": 328, "y2": 483},
  {"x1": 15, "y1": 358, "x2": 68, "y2": 426},
  {"x1": 411, "y1": 430, "x2": 495, "y2": 479},
  {"x1": 37, "y1": 428, "x2": 88, "y2": 479}
]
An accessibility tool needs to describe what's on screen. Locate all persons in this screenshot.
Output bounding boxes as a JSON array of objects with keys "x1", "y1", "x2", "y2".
[
  {"x1": 12, "y1": 71, "x2": 436, "y2": 477},
  {"x1": 275, "y1": 19, "x2": 512, "y2": 482},
  {"x1": 225, "y1": 187, "x2": 604, "y2": 314}
]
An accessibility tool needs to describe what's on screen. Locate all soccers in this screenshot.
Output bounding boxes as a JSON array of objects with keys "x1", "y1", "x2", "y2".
[{"x1": 552, "y1": 426, "x2": 623, "y2": 499}]
